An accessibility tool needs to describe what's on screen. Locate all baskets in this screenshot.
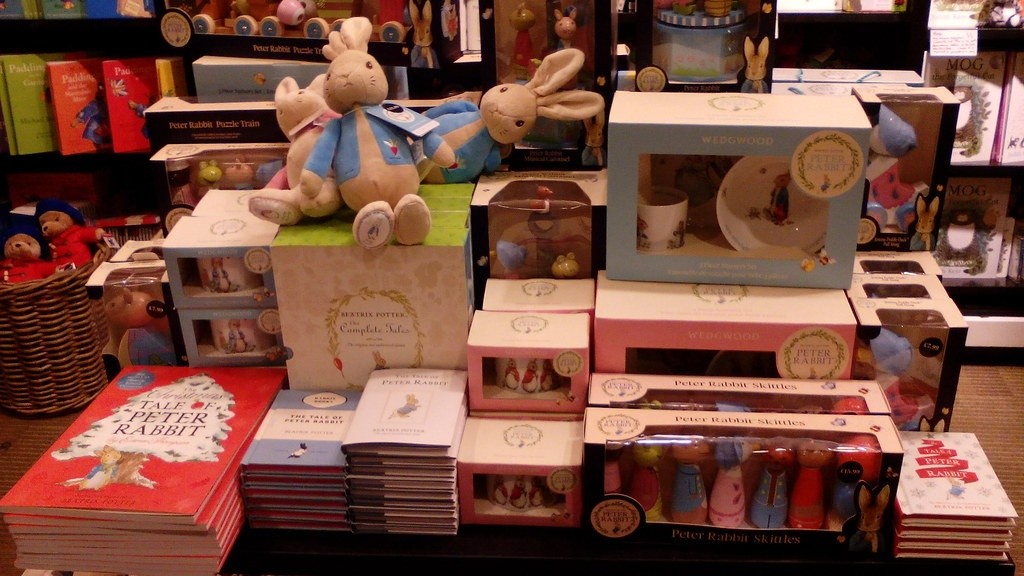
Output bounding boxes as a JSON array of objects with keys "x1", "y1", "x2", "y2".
[{"x1": 0, "y1": 242, "x2": 110, "y2": 415}]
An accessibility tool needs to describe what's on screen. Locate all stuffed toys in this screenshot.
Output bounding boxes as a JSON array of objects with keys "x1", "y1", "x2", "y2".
[
  {"x1": 0, "y1": 198, "x2": 107, "y2": 283},
  {"x1": 248, "y1": 17, "x2": 605, "y2": 248}
]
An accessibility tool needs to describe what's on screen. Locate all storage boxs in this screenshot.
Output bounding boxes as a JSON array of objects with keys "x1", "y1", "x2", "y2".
[{"x1": 0, "y1": 0, "x2": 1024, "y2": 576}]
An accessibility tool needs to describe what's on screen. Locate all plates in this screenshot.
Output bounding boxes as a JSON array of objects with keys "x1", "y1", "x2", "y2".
[{"x1": 716, "y1": 155, "x2": 829, "y2": 257}]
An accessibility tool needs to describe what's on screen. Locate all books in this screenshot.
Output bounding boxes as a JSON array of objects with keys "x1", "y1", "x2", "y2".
[
  {"x1": 922, "y1": 51, "x2": 1024, "y2": 282},
  {"x1": 0, "y1": 365, "x2": 470, "y2": 576},
  {"x1": 892, "y1": 430, "x2": 1018, "y2": 560},
  {"x1": 0, "y1": 0, "x2": 189, "y2": 156}
]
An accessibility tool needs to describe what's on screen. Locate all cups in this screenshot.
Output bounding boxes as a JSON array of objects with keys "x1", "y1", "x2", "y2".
[{"x1": 637, "y1": 184, "x2": 687, "y2": 251}]
